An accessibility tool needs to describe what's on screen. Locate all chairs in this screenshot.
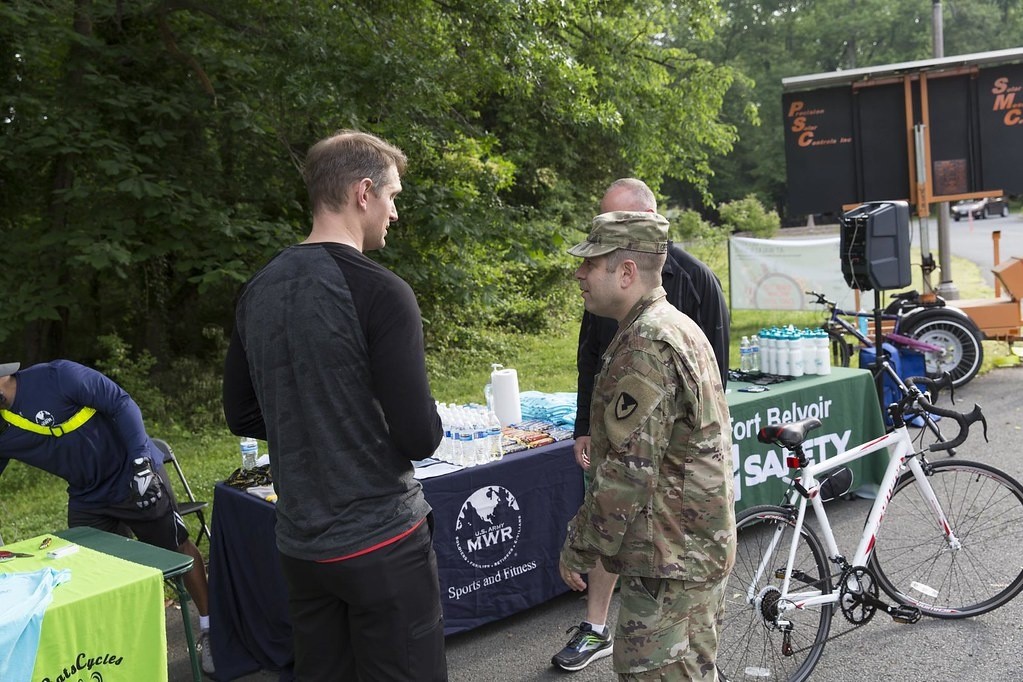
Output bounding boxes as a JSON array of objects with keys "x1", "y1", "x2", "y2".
[{"x1": 126, "y1": 437, "x2": 211, "y2": 591}]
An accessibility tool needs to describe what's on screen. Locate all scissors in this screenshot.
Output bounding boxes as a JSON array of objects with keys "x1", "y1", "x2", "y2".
[{"x1": 0, "y1": 550, "x2": 35, "y2": 558}]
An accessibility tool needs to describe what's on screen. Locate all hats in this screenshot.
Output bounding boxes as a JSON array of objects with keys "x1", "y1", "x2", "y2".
[{"x1": 567, "y1": 210, "x2": 671, "y2": 258}]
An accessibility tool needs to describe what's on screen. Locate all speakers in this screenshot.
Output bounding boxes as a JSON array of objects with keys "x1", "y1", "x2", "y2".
[{"x1": 840, "y1": 200, "x2": 912, "y2": 291}]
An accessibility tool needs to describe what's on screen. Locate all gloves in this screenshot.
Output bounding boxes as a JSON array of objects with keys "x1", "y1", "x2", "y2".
[{"x1": 129, "y1": 468, "x2": 165, "y2": 509}]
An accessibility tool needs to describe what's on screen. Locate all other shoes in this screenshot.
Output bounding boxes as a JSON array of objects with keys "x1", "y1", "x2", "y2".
[{"x1": 199, "y1": 628, "x2": 218, "y2": 674}]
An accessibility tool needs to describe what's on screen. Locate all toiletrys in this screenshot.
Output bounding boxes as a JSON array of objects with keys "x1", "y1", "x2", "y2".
[{"x1": 484, "y1": 363, "x2": 504, "y2": 411}]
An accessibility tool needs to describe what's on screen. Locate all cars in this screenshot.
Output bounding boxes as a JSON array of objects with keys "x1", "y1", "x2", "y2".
[{"x1": 951, "y1": 195, "x2": 1009, "y2": 222}]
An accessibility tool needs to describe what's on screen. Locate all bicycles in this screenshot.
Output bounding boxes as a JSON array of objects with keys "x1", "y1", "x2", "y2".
[
  {"x1": 714, "y1": 369, "x2": 1023, "y2": 682},
  {"x1": 805, "y1": 290, "x2": 984, "y2": 391}
]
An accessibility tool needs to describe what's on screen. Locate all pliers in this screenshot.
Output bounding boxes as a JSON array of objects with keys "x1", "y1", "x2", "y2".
[{"x1": 39, "y1": 538, "x2": 52, "y2": 550}]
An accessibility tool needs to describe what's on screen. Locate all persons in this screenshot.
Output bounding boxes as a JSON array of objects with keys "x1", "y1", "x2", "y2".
[
  {"x1": 0, "y1": 359, "x2": 215, "y2": 682},
  {"x1": 222, "y1": 129, "x2": 449, "y2": 682},
  {"x1": 558, "y1": 212, "x2": 738, "y2": 682},
  {"x1": 552, "y1": 178, "x2": 729, "y2": 670}
]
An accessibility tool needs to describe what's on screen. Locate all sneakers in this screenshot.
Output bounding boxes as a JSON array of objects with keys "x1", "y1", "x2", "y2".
[{"x1": 551, "y1": 621, "x2": 614, "y2": 672}]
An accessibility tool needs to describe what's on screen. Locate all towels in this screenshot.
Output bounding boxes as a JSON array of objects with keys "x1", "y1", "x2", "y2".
[{"x1": 0, "y1": 567, "x2": 73, "y2": 682}]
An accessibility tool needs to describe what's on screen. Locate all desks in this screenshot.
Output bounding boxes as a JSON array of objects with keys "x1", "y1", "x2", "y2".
[
  {"x1": 198, "y1": 436, "x2": 584, "y2": 682},
  {"x1": 724, "y1": 365, "x2": 890, "y2": 530},
  {"x1": 0, "y1": 525, "x2": 202, "y2": 682}
]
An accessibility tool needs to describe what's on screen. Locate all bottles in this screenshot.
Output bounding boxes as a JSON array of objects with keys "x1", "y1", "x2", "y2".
[
  {"x1": 240, "y1": 437, "x2": 258, "y2": 470},
  {"x1": 757, "y1": 325, "x2": 831, "y2": 377},
  {"x1": 740, "y1": 335, "x2": 761, "y2": 372},
  {"x1": 431, "y1": 381, "x2": 504, "y2": 468}
]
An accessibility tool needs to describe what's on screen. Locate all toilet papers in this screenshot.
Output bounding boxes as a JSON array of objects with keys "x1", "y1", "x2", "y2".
[{"x1": 490, "y1": 368, "x2": 523, "y2": 427}]
